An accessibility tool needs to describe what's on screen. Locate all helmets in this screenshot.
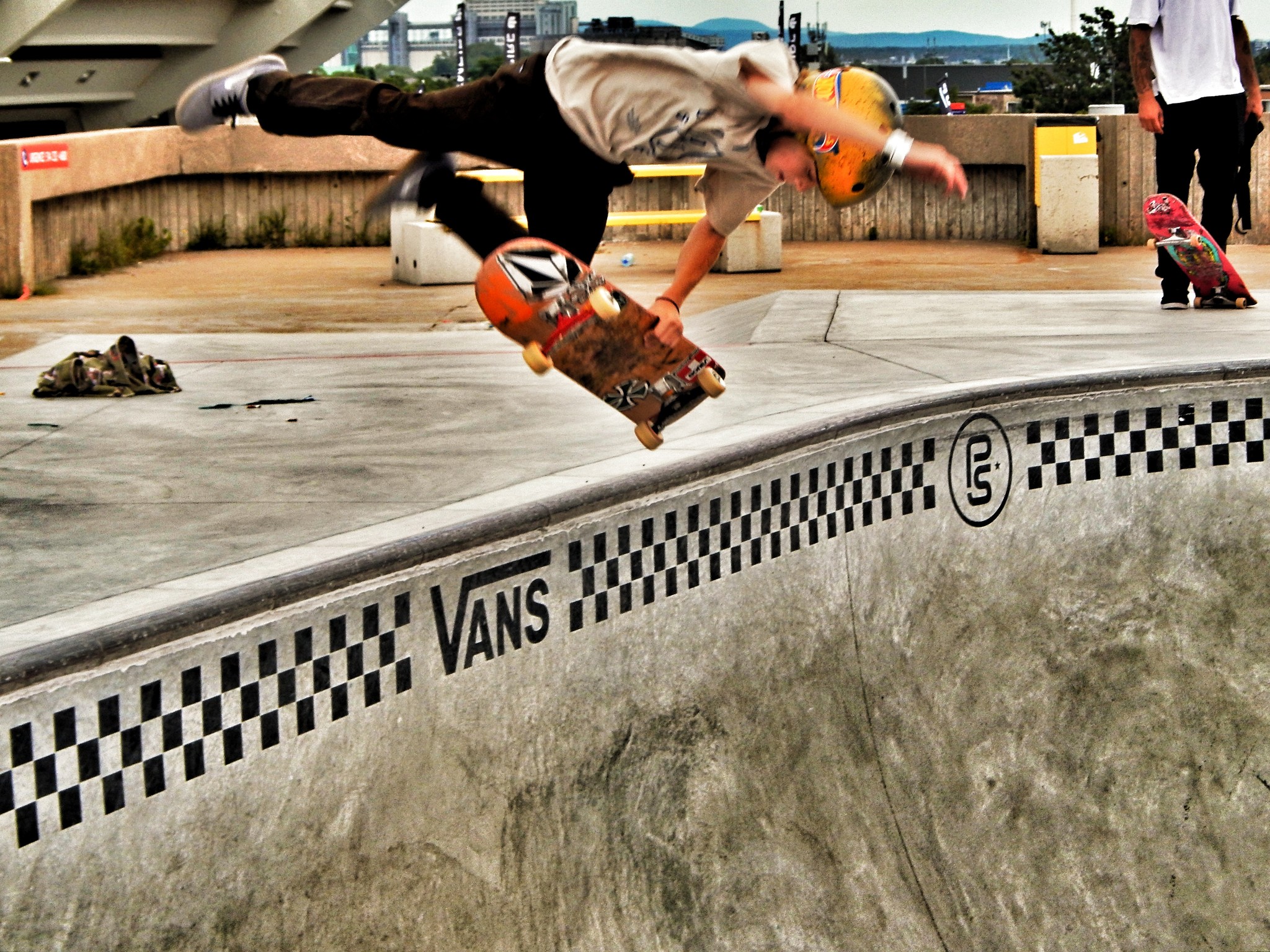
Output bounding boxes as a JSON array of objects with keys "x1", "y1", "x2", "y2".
[{"x1": 799, "y1": 64, "x2": 904, "y2": 212}]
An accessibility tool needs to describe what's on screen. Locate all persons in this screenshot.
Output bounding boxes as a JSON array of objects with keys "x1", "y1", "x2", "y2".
[
  {"x1": 178, "y1": 36, "x2": 974, "y2": 355},
  {"x1": 1127, "y1": 0, "x2": 1265, "y2": 310}
]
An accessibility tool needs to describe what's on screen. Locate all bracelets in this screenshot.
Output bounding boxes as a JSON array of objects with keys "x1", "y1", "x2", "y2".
[
  {"x1": 655, "y1": 296, "x2": 680, "y2": 315},
  {"x1": 877, "y1": 128, "x2": 913, "y2": 170}
]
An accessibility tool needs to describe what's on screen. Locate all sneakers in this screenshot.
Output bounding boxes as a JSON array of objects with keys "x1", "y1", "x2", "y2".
[
  {"x1": 359, "y1": 151, "x2": 454, "y2": 224},
  {"x1": 177, "y1": 55, "x2": 288, "y2": 139}
]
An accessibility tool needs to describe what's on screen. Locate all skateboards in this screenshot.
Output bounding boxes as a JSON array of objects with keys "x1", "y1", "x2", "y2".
[
  {"x1": 475, "y1": 238, "x2": 727, "y2": 451},
  {"x1": 1145, "y1": 193, "x2": 1258, "y2": 309}
]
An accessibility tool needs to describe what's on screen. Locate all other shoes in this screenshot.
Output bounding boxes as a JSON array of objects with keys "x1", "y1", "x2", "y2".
[{"x1": 1160, "y1": 289, "x2": 1192, "y2": 311}]
